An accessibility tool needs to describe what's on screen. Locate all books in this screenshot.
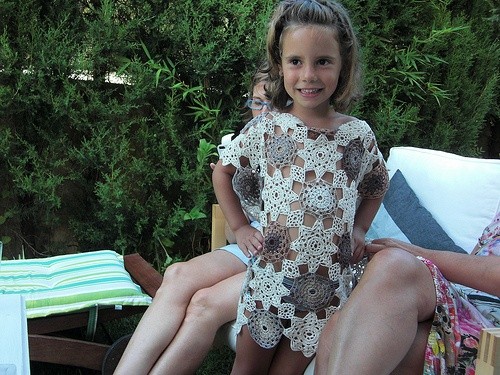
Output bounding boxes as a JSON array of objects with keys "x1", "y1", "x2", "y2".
[{"x1": 475, "y1": 327, "x2": 500, "y2": 375}]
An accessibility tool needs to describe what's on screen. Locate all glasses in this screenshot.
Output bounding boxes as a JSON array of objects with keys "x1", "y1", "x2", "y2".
[{"x1": 247, "y1": 100, "x2": 278, "y2": 111}]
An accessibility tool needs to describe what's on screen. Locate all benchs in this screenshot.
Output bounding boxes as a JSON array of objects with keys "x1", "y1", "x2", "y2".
[{"x1": 211, "y1": 147, "x2": 500, "y2": 375}]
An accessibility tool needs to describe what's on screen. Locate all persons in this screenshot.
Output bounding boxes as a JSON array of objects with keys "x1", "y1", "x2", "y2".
[
  {"x1": 313, "y1": 200, "x2": 500, "y2": 375},
  {"x1": 112, "y1": 60, "x2": 275, "y2": 375},
  {"x1": 212, "y1": 12, "x2": 390, "y2": 375}
]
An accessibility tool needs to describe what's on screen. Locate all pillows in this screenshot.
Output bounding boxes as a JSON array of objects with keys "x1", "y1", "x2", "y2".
[{"x1": 0, "y1": 250, "x2": 153, "y2": 319}]
